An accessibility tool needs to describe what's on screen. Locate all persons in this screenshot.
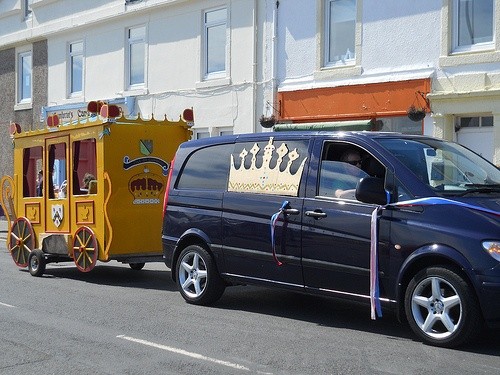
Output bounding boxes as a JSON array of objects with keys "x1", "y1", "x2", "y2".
[
  {"x1": 36, "y1": 168, "x2": 97, "y2": 199},
  {"x1": 331, "y1": 150, "x2": 363, "y2": 199}
]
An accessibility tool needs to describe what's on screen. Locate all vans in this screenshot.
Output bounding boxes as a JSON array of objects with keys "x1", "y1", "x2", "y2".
[{"x1": 161, "y1": 130, "x2": 500, "y2": 348}]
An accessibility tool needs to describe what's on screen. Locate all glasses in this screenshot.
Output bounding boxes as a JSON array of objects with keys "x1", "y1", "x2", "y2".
[{"x1": 344, "y1": 160, "x2": 363, "y2": 166}]
